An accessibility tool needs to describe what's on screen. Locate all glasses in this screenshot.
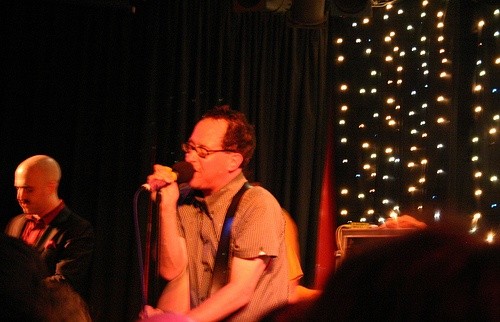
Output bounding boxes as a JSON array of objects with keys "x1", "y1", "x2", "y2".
[{"x1": 181, "y1": 142, "x2": 236, "y2": 158}]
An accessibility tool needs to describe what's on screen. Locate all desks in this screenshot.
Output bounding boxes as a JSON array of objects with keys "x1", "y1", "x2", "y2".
[{"x1": 334, "y1": 224, "x2": 427, "y2": 272}]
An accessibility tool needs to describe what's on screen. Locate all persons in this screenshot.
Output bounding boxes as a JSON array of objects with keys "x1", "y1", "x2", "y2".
[
  {"x1": 5, "y1": 155, "x2": 94, "y2": 308},
  {"x1": 138, "y1": 105, "x2": 291, "y2": 322},
  {"x1": 1, "y1": 234, "x2": 92, "y2": 322}
]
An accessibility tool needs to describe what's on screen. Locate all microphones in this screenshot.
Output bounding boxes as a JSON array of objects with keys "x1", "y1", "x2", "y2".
[{"x1": 142, "y1": 161, "x2": 194, "y2": 191}]
